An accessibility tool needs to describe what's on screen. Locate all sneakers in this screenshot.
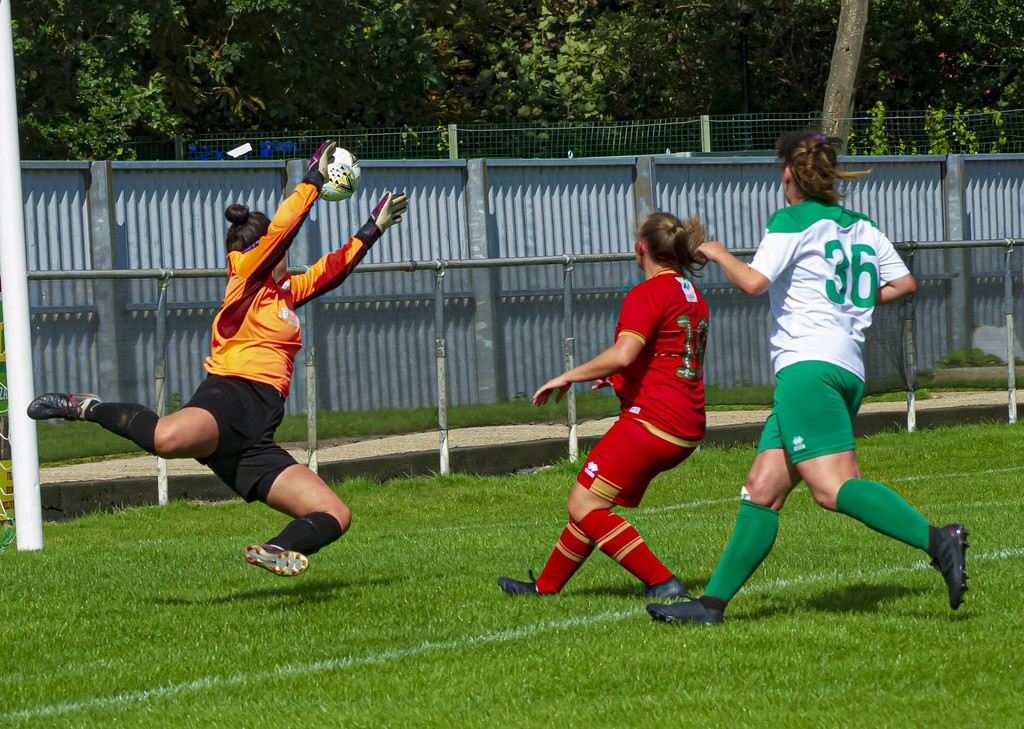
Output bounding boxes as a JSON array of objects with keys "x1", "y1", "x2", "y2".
[
  {"x1": 498, "y1": 569, "x2": 542, "y2": 597},
  {"x1": 641, "y1": 574, "x2": 689, "y2": 600},
  {"x1": 646, "y1": 594, "x2": 728, "y2": 626},
  {"x1": 929, "y1": 524, "x2": 970, "y2": 610},
  {"x1": 245, "y1": 544, "x2": 309, "y2": 575},
  {"x1": 27, "y1": 393, "x2": 104, "y2": 421}
]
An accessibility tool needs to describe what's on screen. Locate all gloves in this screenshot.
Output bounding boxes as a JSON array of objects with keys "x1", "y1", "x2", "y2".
[
  {"x1": 303, "y1": 139, "x2": 336, "y2": 190},
  {"x1": 360, "y1": 191, "x2": 409, "y2": 241}
]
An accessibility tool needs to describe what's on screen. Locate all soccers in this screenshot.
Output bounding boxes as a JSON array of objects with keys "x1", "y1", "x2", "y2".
[{"x1": 317, "y1": 146, "x2": 362, "y2": 202}]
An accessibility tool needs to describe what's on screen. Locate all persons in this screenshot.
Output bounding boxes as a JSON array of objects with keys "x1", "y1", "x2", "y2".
[
  {"x1": 498, "y1": 213, "x2": 713, "y2": 599},
  {"x1": 646, "y1": 134, "x2": 970, "y2": 624},
  {"x1": 25, "y1": 140, "x2": 406, "y2": 576}
]
